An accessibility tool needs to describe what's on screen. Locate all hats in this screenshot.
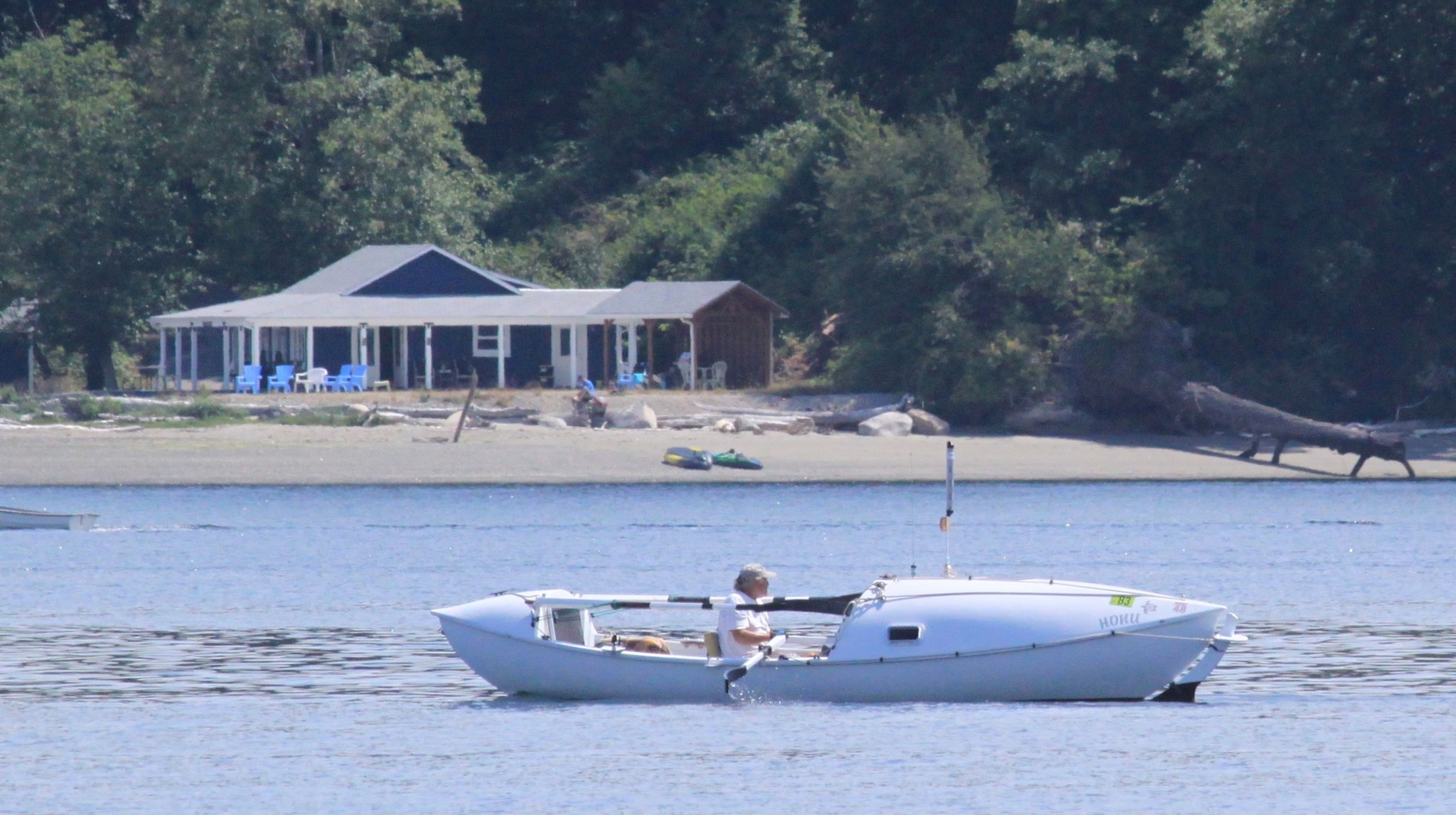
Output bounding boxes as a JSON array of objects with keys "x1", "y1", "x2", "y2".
[{"x1": 734, "y1": 563, "x2": 776, "y2": 589}]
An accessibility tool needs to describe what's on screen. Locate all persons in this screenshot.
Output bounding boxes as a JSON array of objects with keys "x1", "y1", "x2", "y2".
[
  {"x1": 572, "y1": 373, "x2": 595, "y2": 408},
  {"x1": 717, "y1": 564, "x2": 808, "y2": 659}
]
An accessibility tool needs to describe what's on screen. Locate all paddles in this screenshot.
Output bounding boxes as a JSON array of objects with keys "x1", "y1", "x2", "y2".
[{"x1": 609, "y1": 593, "x2": 863, "y2": 619}]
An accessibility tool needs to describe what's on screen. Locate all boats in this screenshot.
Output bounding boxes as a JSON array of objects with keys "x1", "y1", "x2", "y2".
[
  {"x1": 709, "y1": 449, "x2": 764, "y2": 470},
  {"x1": 423, "y1": 571, "x2": 1252, "y2": 705},
  {"x1": 856, "y1": 409, "x2": 914, "y2": 437},
  {"x1": 665, "y1": 446, "x2": 714, "y2": 471},
  {"x1": 907, "y1": 405, "x2": 951, "y2": 437}
]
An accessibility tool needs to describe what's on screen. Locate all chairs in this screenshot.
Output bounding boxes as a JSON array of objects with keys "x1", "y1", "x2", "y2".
[
  {"x1": 702, "y1": 630, "x2": 722, "y2": 657},
  {"x1": 675, "y1": 359, "x2": 702, "y2": 389},
  {"x1": 235, "y1": 365, "x2": 264, "y2": 395},
  {"x1": 702, "y1": 360, "x2": 729, "y2": 391},
  {"x1": 619, "y1": 361, "x2": 647, "y2": 391},
  {"x1": 452, "y1": 366, "x2": 473, "y2": 386},
  {"x1": 293, "y1": 367, "x2": 328, "y2": 394},
  {"x1": 411, "y1": 359, "x2": 435, "y2": 389},
  {"x1": 267, "y1": 364, "x2": 296, "y2": 394},
  {"x1": 336, "y1": 363, "x2": 368, "y2": 392},
  {"x1": 323, "y1": 364, "x2": 354, "y2": 392}
]
